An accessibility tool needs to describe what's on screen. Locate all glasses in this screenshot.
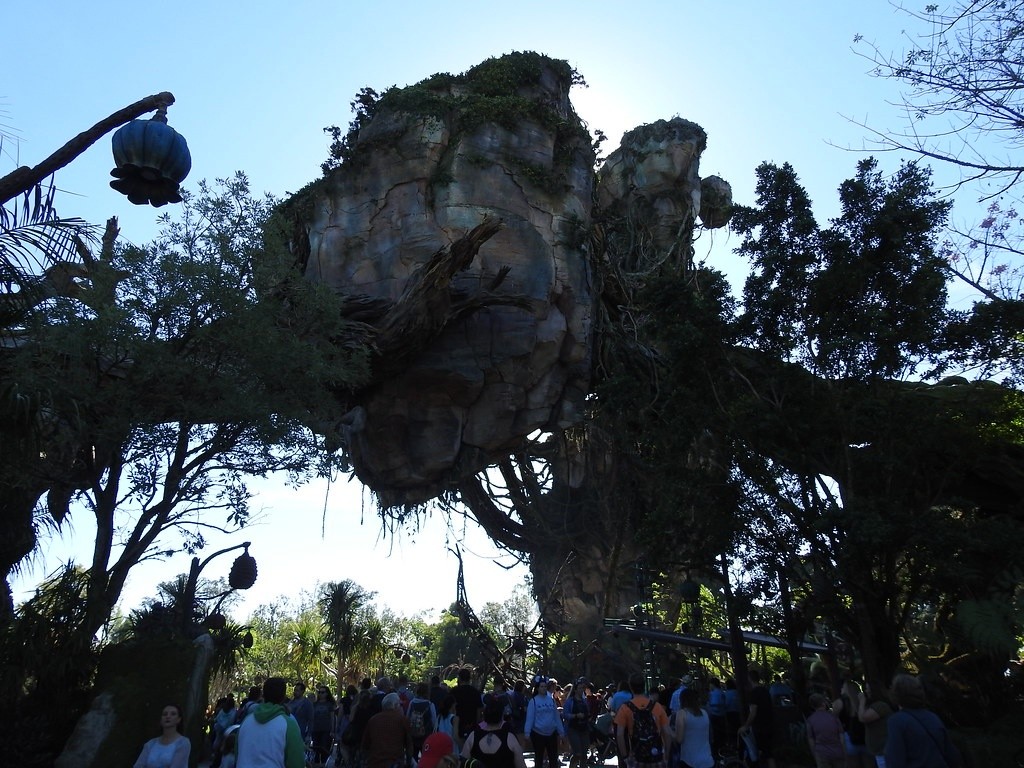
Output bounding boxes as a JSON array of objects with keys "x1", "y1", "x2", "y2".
[{"x1": 318, "y1": 691, "x2": 326, "y2": 694}]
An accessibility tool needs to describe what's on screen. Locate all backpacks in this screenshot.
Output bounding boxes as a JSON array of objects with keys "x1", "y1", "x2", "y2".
[
  {"x1": 623, "y1": 698, "x2": 664, "y2": 763},
  {"x1": 408, "y1": 702, "x2": 431, "y2": 735},
  {"x1": 234, "y1": 703, "x2": 255, "y2": 725},
  {"x1": 399, "y1": 688, "x2": 409, "y2": 713}
]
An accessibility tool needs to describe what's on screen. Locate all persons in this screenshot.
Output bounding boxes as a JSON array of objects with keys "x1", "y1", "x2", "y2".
[
  {"x1": 132, "y1": 703, "x2": 191, "y2": 768},
  {"x1": 208, "y1": 651, "x2": 962, "y2": 768}
]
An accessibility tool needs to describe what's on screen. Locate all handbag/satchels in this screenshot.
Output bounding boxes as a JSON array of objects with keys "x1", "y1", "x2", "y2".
[{"x1": 741, "y1": 725, "x2": 759, "y2": 762}]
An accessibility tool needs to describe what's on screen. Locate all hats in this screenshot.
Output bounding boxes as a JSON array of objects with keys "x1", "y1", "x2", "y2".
[
  {"x1": 418, "y1": 732, "x2": 453, "y2": 768},
  {"x1": 681, "y1": 674, "x2": 695, "y2": 686}
]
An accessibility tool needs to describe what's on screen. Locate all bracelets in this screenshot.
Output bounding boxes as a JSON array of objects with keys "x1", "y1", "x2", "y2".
[
  {"x1": 623, "y1": 752, "x2": 629, "y2": 759},
  {"x1": 575, "y1": 713, "x2": 577, "y2": 719}
]
